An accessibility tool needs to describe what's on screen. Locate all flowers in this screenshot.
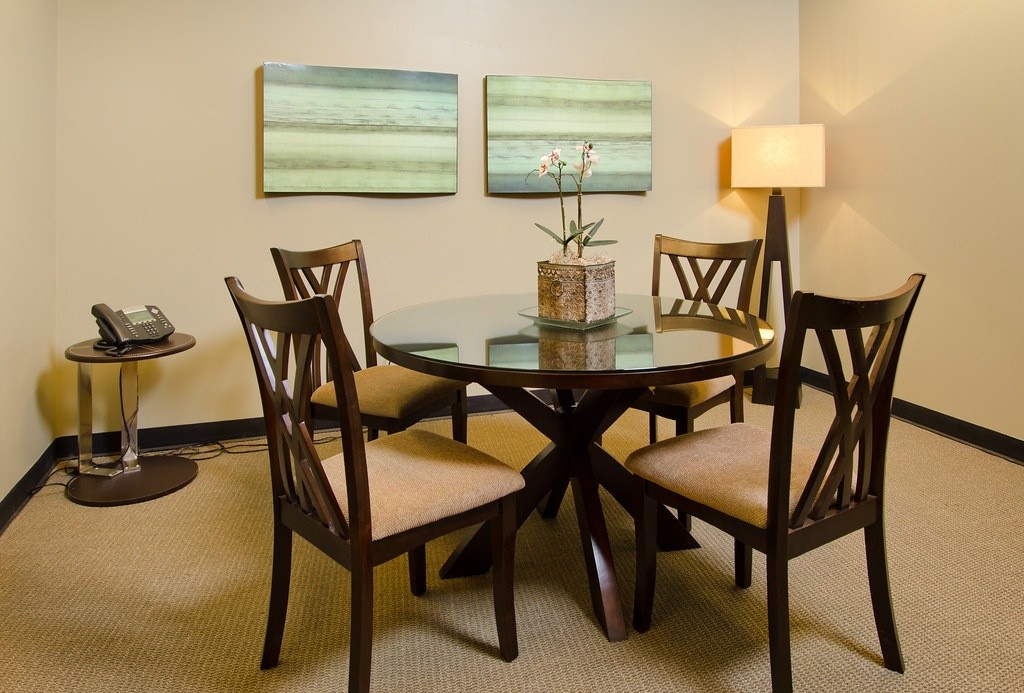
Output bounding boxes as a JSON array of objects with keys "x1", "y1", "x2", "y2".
[{"x1": 523, "y1": 139, "x2": 618, "y2": 257}]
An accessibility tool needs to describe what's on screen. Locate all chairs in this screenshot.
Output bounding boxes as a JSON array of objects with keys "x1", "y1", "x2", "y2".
[
  {"x1": 271, "y1": 239, "x2": 473, "y2": 446},
  {"x1": 629, "y1": 234, "x2": 763, "y2": 531},
  {"x1": 625, "y1": 272, "x2": 926, "y2": 693},
  {"x1": 224, "y1": 276, "x2": 526, "y2": 693}
]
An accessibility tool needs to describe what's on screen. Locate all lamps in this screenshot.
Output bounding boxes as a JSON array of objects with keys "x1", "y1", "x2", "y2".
[{"x1": 729, "y1": 123, "x2": 827, "y2": 409}]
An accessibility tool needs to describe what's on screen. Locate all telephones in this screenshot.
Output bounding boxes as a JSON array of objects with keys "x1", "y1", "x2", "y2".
[{"x1": 91, "y1": 302, "x2": 175, "y2": 348}]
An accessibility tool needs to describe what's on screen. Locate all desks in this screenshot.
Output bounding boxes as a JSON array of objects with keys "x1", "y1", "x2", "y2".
[
  {"x1": 368, "y1": 291, "x2": 777, "y2": 643},
  {"x1": 65, "y1": 332, "x2": 197, "y2": 508}
]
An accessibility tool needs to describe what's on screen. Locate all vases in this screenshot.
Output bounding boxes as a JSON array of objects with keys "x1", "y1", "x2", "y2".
[
  {"x1": 537, "y1": 260, "x2": 617, "y2": 323},
  {"x1": 539, "y1": 326, "x2": 616, "y2": 372}
]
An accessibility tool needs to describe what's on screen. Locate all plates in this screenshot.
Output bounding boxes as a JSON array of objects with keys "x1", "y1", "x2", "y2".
[
  {"x1": 520, "y1": 306, "x2": 633, "y2": 327},
  {"x1": 522, "y1": 322, "x2": 634, "y2": 341}
]
